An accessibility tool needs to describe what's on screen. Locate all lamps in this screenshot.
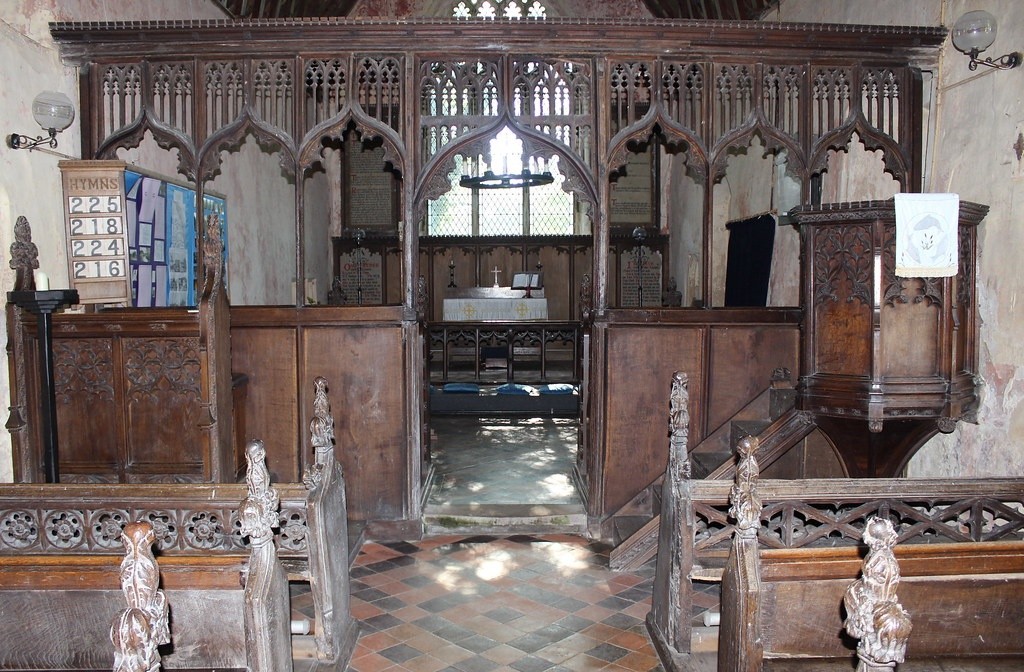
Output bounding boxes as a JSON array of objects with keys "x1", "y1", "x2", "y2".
[
  {"x1": 11, "y1": 89, "x2": 74, "y2": 153},
  {"x1": 952, "y1": 10, "x2": 1023, "y2": 72}
]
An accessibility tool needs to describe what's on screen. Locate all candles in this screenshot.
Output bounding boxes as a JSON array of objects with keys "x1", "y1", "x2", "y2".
[{"x1": 36, "y1": 272, "x2": 48, "y2": 291}]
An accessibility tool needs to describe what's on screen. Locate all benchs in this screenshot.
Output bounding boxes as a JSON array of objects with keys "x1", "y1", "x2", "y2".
[
  {"x1": 644, "y1": 369, "x2": 1024, "y2": 672},
  {"x1": 0, "y1": 375, "x2": 360, "y2": 672}
]
